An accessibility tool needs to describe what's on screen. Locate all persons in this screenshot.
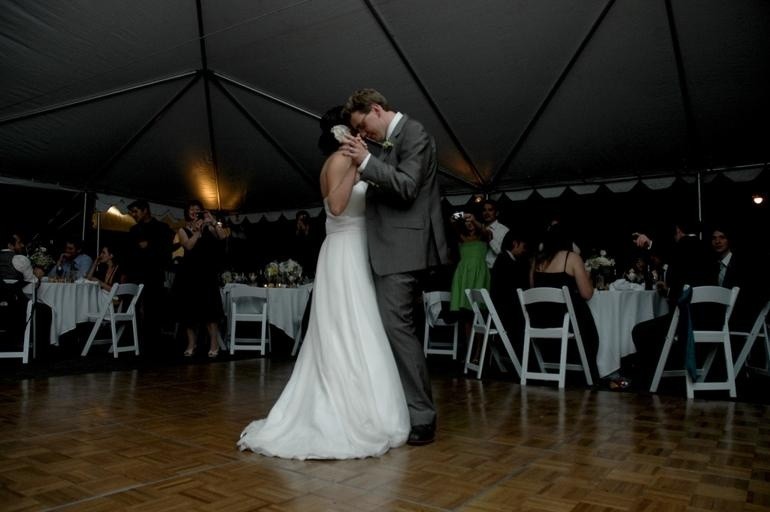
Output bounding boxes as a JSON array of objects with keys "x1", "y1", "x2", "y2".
[
  {"x1": 340, "y1": 88, "x2": 448, "y2": 445},
  {"x1": 236, "y1": 106, "x2": 411, "y2": 462},
  {"x1": 0, "y1": 199, "x2": 325, "y2": 358},
  {"x1": 447, "y1": 200, "x2": 742, "y2": 400}
]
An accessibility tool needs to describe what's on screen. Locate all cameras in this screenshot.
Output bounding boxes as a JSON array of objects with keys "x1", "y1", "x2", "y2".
[
  {"x1": 300, "y1": 213, "x2": 311, "y2": 225},
  {"x1": 195, "y1": 211, "x2": 205, "y2": 222},
  {"x1": 453, "y1": 211, "x2": 466, "y2": 220}
]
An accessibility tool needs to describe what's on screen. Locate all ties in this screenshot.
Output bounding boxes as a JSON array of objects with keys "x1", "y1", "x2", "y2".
[{"x1": 719, "y1": 263, "x2": 724, "y2": 285}]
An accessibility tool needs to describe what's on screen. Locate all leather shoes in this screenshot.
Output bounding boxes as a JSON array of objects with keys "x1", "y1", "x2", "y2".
[{"x1": 408, "y1": 415, "x2": 436, "y2": 446}]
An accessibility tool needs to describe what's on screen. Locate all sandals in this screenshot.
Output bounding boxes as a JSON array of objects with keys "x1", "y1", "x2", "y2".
[
  {"x1": 184, "y1": 345, "x2": 196, "y2": 356},
  {"x1": 208, "y1": 346, "x2": 218, "y2": 357}
]
